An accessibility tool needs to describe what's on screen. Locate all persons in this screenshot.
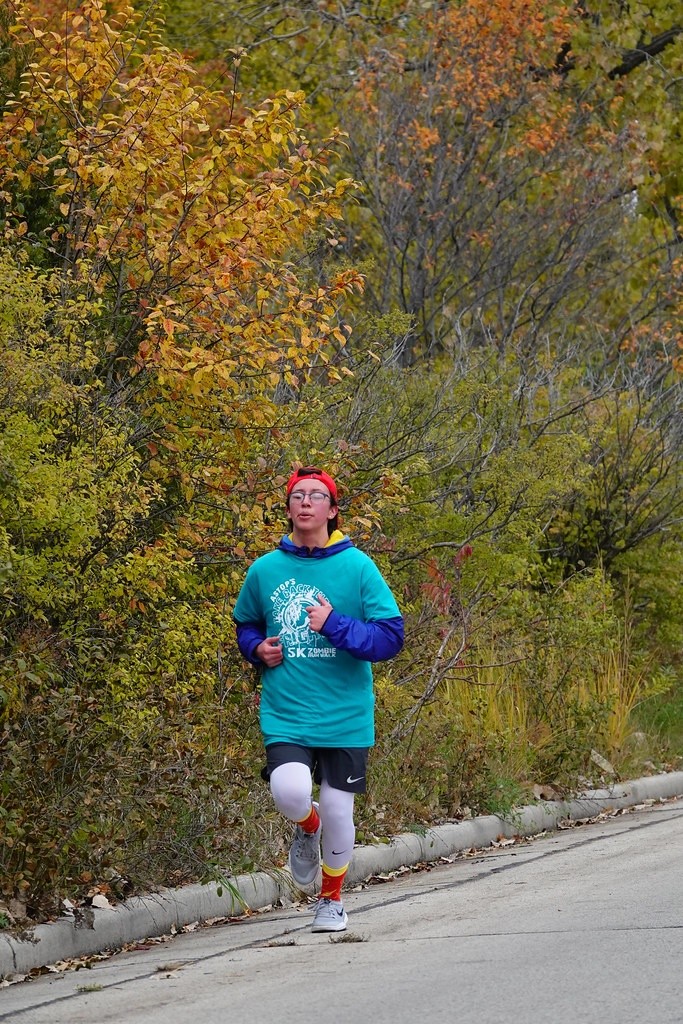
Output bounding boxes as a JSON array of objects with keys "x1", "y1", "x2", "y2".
[{"x1": 230, "y1": 467, "x2": 404, "y2": 933}]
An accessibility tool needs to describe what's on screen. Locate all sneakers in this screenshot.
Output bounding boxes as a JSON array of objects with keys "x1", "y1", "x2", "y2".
[
  {"x1": 289, "y1": 801, "x2": 322, "y2": 885},
  {"x1": 311, "y1": 898, "x2": 348, "y2": 932}
]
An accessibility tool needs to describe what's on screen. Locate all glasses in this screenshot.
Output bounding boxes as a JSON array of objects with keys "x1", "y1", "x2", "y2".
[{"x1": 288, "y1": 491, "x2": 331, "y2": 504}]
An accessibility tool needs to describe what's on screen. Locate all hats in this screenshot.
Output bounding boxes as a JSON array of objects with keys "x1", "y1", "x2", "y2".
[{"x1": 287, "y1": 466, "x2": 337, "y2": 503}]
